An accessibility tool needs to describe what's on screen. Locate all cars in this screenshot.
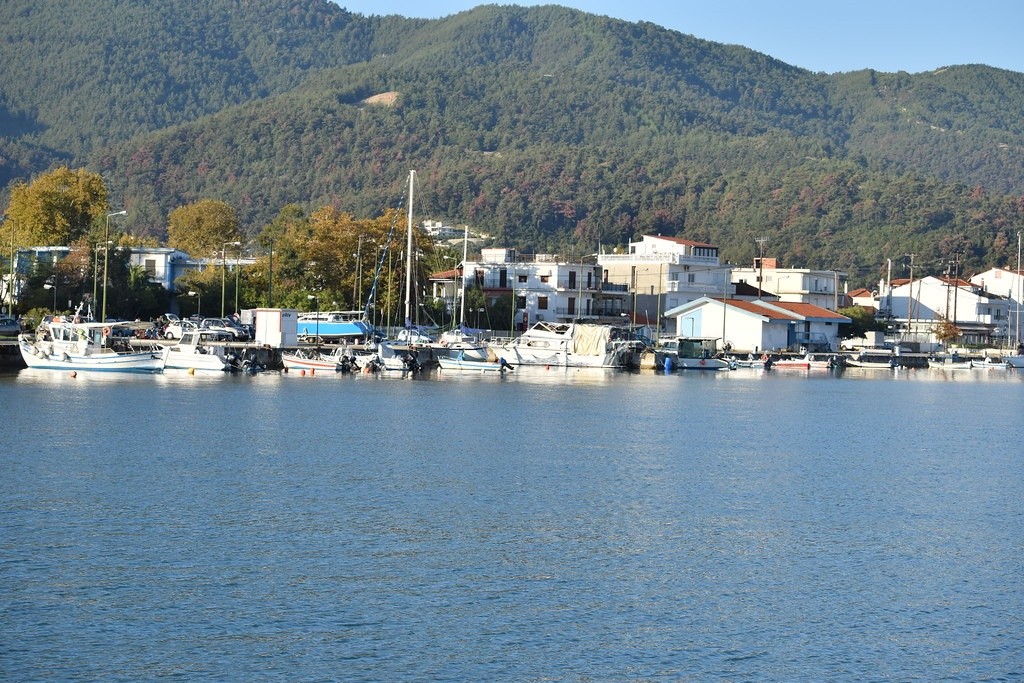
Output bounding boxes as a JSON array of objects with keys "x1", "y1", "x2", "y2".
[
  {"x1": 40, "y1": 312, "x2": 124, "y2": 325},
  {"x1": 396, "y1": 329, "x2": 432, "y2": 345},
  {"x1": 159, "y1": 313, "x2": 255, "y2": 341},
  {"x1": 0, "y1": 314, "x2": 21, "y2": 338}
]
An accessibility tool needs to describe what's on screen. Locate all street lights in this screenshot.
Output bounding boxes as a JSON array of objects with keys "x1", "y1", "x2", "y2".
[
  {"x1": 307, "y1": 294, "x2": 321, "y2": 345},
  {"x1": 579, "y1": 253, "x2": 599, "y2": 321},
  {"x1": 91, "y1": 241, "x2": 114, "y2": 319},
  {"x1": 221, "y1": 241, "x2": 242, "y2": 319},
  {"x1": 101, "y1": 209, "x2": 127, "y2": 325},
  {"x1": 886, "y1": 257, "x2": 892, "y2": 292}
]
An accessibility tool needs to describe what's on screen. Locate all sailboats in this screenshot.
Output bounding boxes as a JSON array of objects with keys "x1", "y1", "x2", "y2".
[
  {"x1": 341, "y1": 169, "x2": 500, "y2": 362},
  {"x1": 1003, "y1": 231, "x2": 1024, "y2": 373}
]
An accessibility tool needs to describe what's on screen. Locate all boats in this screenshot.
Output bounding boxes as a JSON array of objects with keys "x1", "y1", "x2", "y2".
[
  {"x1": 475, "y1": 316, "x2": 664, "y2": 381},
  {"x1": 973, "y1": 358, "x2": 1008, "y2": 372},
  {"x1": 927, "y1": 357, "x2": 972, "y2": 374},
  {"x1": 438, "y1": 357, "x2": 512, "y2": 376},
  {"x1": 671, "y1": 336, "x2": 931, "y2": 375},
  {"x1": 298, "y1": 310, "x2": 378, "y2": 345},
  {"x1": 17, "y1": 322, "x2": 375, "y2": 377}
]
